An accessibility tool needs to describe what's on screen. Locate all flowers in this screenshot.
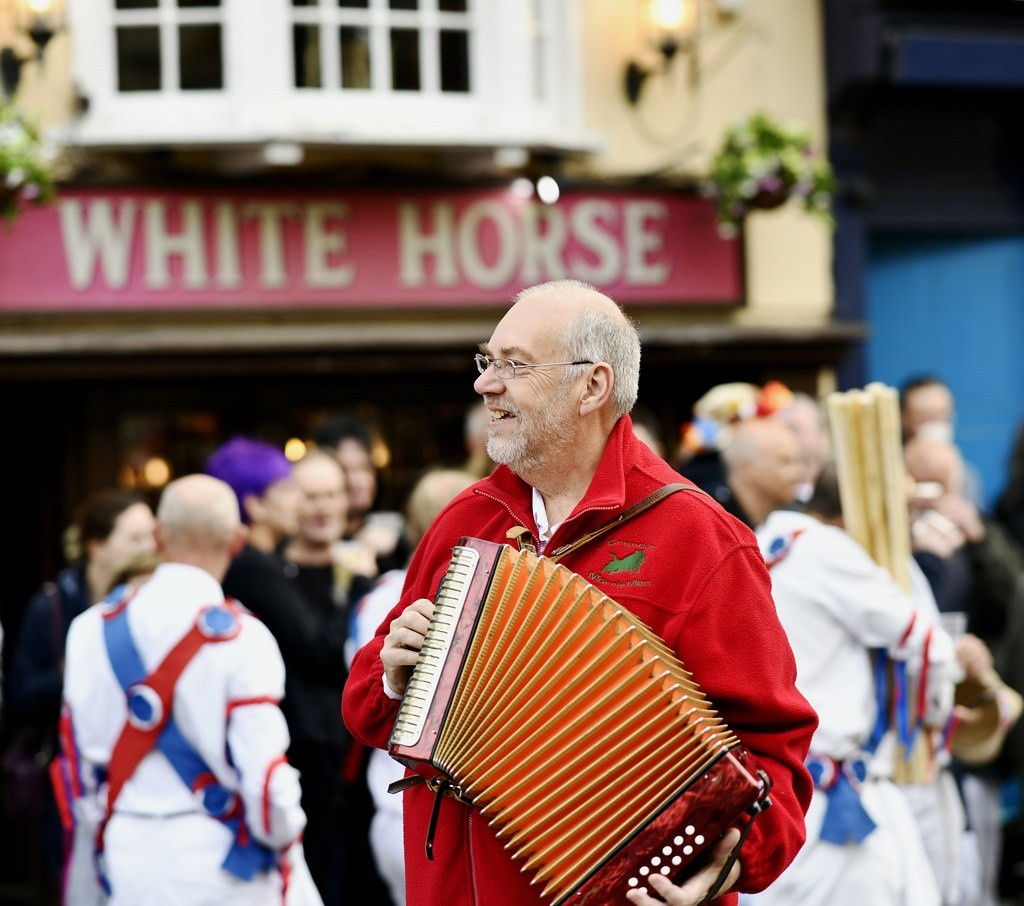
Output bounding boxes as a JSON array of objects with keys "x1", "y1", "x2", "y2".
[
  {"x1": 703, "y1": 122, "x2": 842, "y2": 242},
  {"x1": 0, "y1": 101, "x2": 83, "y2": 219}
]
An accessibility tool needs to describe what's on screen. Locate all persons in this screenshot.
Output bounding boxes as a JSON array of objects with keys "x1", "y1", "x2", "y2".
[
  {"x1": 63, "y1": 475, "x2": 325, "y2": 905},
  {"x1": 12, "y1": 372, "x2": 1023, "y2": 905},
  {"x1": 341, "y1": 279, "x2": 819, "y2": 905}
]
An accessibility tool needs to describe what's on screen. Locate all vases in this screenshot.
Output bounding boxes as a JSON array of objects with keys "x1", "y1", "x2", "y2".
[{"x1": 737, "y1": 164, "x2": 797, "y2": 212}]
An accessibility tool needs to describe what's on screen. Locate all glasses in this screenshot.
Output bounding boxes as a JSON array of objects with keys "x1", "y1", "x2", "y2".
[{"x1": 474, "y1": 353, "x2": 596, "y2": 379}]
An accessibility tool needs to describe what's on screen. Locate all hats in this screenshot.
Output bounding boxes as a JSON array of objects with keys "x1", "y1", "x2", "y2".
[{"x1": 211, "y1": 434, "x2": 295, "y2": 510}]
